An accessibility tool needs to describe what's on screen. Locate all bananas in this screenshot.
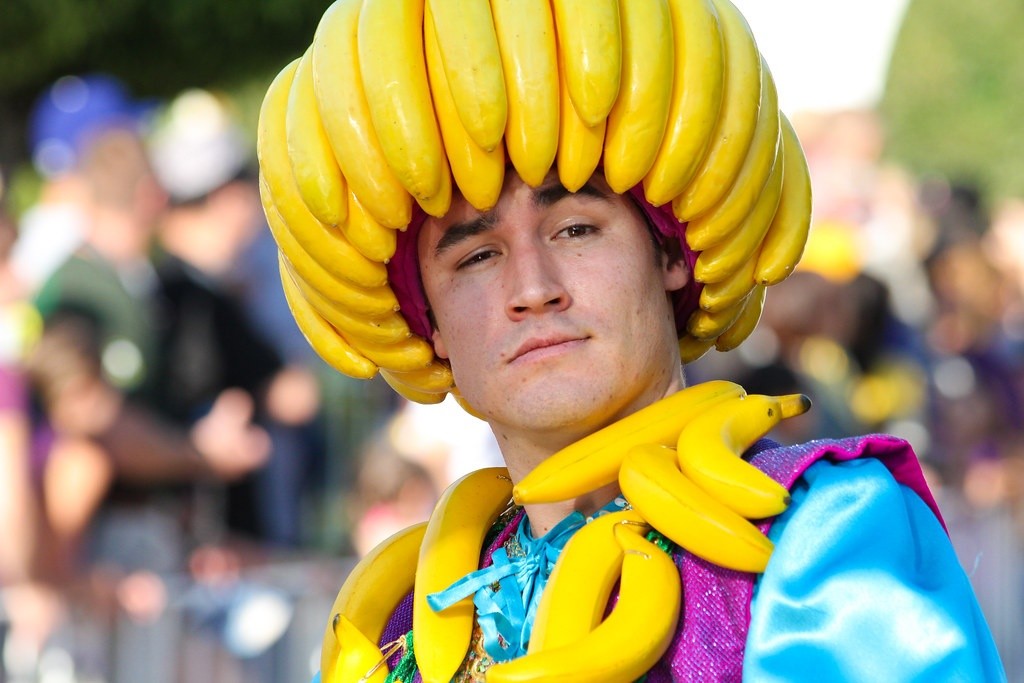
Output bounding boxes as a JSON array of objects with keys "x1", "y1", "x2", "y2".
[{"x1": 317, "y1": 378, "x2": 811, "y2": 683}]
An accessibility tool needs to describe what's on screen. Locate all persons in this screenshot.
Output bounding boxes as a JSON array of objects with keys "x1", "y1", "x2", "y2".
[
  {"x1": 312, "y1": 167, "x2": 1006, "y2": 683},
  {"x1": 683, "y1": 164, "x2": 1024, "y2": 683},
  {"x1": 0, "y1": 85, "x2": 329, "y2": 683}
]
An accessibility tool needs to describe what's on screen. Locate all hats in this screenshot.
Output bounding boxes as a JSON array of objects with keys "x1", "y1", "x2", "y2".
[
  {"x1": 387, "y1": 157, "x2": 705, "y2": 346},
  {"x1": 28, "y1": 74, "x2": 158, "y2": 172},
  {"x1": 148, "y1": 90, "x2": 251, "y2": 200}
]
